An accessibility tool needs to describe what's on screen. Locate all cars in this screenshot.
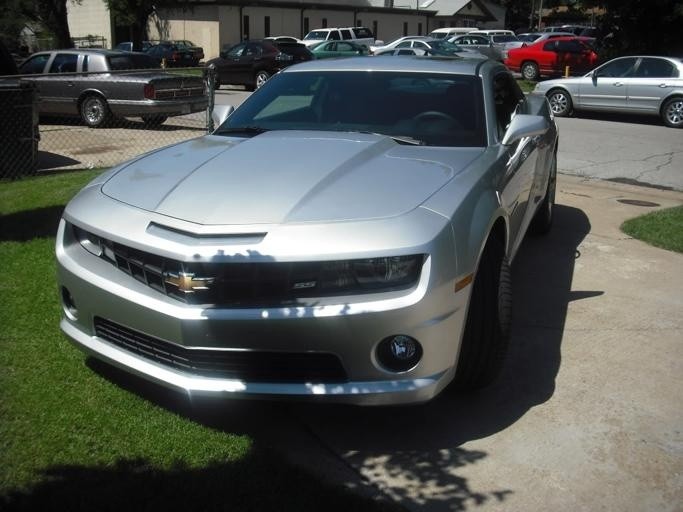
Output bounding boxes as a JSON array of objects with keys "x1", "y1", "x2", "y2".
[
  {"x1": 18, "y1": 41, "x2": 208, "y2": 127},
  {"x1": 534, "y1": 55, "x2": 683, "y2": 127},
  {"x1": 56, "y1": 56, "x2": 559, "y2": 407}
]
